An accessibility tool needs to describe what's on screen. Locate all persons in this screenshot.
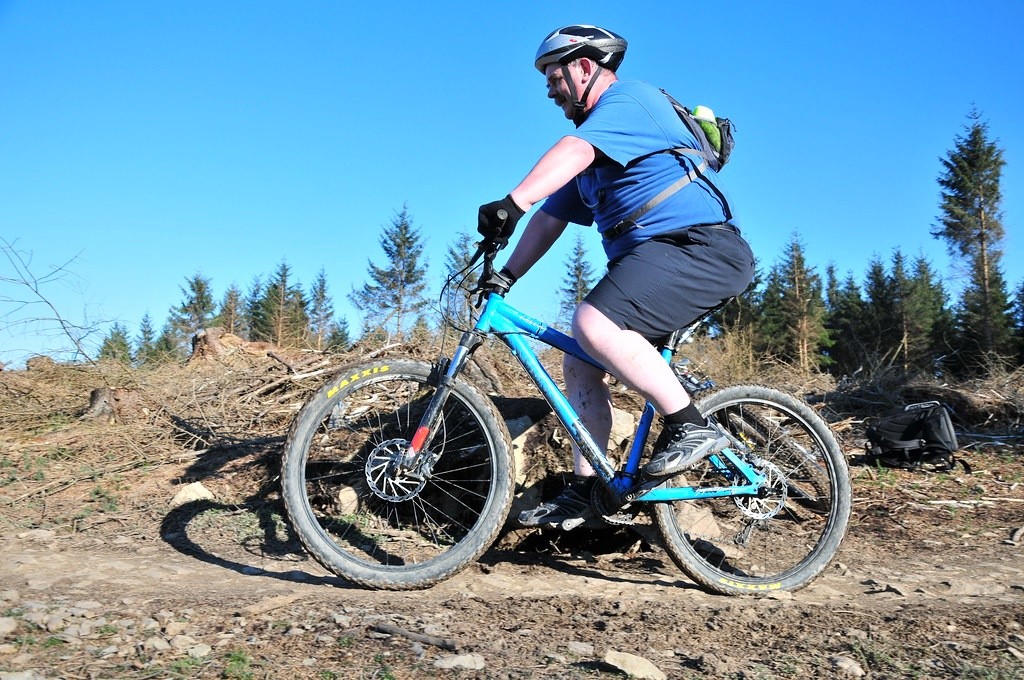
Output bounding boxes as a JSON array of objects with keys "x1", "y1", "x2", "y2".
[{"x1": 477, "y1": 25, "x2": 755, "y2": 527}]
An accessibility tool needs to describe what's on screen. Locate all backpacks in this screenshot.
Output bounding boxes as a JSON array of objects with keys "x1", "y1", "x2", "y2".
[{"x1": 598, "y1": 88, "x2": 737, "y2": 241}]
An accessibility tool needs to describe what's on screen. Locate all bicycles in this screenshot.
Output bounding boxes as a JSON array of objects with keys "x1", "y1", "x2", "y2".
[{"x1": 280, "y1": 209, "x2": 853, "y2": 597}]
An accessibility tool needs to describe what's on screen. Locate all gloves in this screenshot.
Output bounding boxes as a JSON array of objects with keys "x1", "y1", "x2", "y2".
[
  {"x1": 477, "y1": 266, "x2": 517, "y2": 300},
  {"x1": 477, "y1": 194, "x2": 526, "y2": 239}
]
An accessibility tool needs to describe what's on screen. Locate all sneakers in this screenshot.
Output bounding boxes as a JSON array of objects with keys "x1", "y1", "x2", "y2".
[
  {"x1": 643, "y1": 416, "x2": 731, "y2": 476},
  {"x1": 518, "y1": 485, "x2": 595, "y2": 526}
]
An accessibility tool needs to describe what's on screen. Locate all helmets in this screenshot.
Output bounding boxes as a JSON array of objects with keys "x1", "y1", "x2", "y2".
[{"x1": 534, "y1": 24, "x2": 628, "y2": 123}]
